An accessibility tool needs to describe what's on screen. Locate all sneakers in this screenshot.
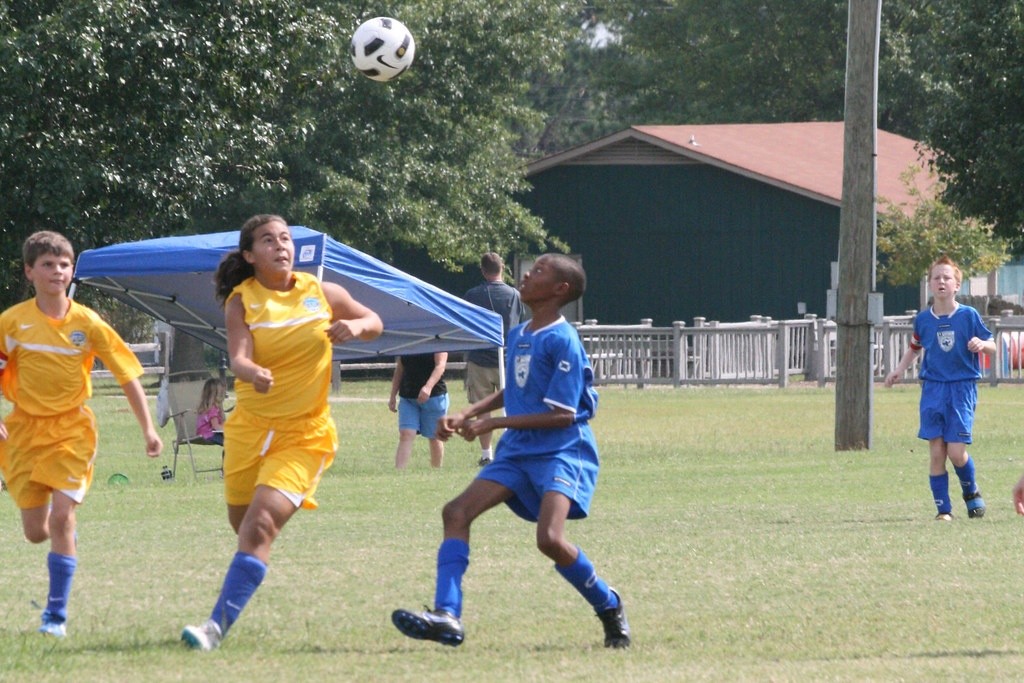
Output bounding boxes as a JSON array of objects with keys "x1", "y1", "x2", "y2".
[
  {"x1": 962, "y1": 482, "x2": 986, "y2": 518},
  {"x1": 391, "y1": 607, "x2": 464, "y2": 648},
  {"x1": 593, "y1": 584, "x2": 631, "y2": 649},
  {"x1": 39, "y1": 621, "x2": 66, "y2": 637},
  {"x1": 935, "y1": 513, "x2": 954, "y2": 521},
  {"x1": 182, "y1": 620, "x2": 222, "y2": 651}
]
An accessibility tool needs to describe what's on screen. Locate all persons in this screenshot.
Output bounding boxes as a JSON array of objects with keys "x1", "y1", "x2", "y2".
[
  {"x1": 388, "y1": 352, "x2": 449, "y2": 468},
  {"x1": 393, "y1": 253, "x2": 630, "y2": 650},
  {"x1": 178, "y1": 214, "x2": 382, "y2": 652},
  {"x1": 2, "y1": 231, "x2": 163, "y2": 635},
  {"x1": 197, "y1": 378, "x2": 226, "y2": 478},
  {"x1": 884, "y1": 255, "x2": 996, "y2": 520},
  {"x1": 1012, "y1": 473, "x2": 1024, "y2": 517},
  {"x1": 460, "y1": 253, "x2": 527, "y2": 466}
]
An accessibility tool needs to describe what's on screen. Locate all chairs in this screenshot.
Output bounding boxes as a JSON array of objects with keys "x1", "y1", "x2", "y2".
[{"x1": 165, "y1": 370, "x2": 228, "y2": 485}]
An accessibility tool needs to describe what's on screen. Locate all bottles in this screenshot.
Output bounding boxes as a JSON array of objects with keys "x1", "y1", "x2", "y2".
[{"x1": 161, "y1": 466, "x2": 173, "y2": 484}]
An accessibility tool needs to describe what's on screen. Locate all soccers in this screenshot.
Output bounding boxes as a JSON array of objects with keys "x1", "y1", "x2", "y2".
[{"x1": 348, "y1": 16, "x2": 418, "y2": 84}]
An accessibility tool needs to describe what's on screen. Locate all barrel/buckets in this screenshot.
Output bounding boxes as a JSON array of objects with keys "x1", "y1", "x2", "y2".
[{"x1": 108, "y1": 474, "x2": 128, "y2": 493}]
